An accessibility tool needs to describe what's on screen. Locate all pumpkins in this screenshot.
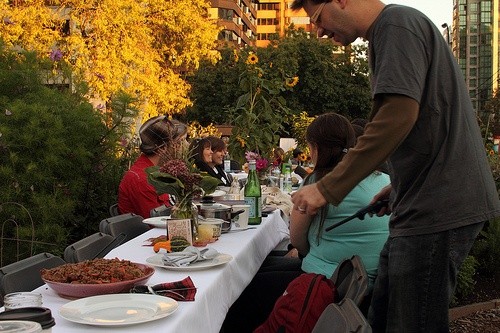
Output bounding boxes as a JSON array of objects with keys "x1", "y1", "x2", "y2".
[{"x1": 153, "y1": 236, "x2": 191, "y2": 253}]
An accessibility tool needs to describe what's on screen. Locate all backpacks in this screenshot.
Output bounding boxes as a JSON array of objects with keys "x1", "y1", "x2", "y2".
[{"x1": 254, "y1": 273, "x2": 337, "y2": 333}]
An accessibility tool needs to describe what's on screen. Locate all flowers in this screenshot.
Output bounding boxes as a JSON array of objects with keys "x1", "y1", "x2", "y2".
[
  {"x1": 270, "y1": 155, "x2": 284, "y2": 170},
  {"x1": 287, "y1": 144, "x2": 311, "y2": 166},
  {"x1": 242, "y1": 151, "x2": 269, "y2": 174},
  {"x1": 145, "y1": 128, "x2": 221, "y2": 238}
]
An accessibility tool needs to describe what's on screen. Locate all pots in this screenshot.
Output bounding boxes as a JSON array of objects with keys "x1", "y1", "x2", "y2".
[{"x1": 195, "y1": 195, "x2": 245, "y2": 232}]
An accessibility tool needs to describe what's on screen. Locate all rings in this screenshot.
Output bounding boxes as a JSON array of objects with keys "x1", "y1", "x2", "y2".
[{"x1": 298, "y1": 206, "x2": 306, "y2": 211}]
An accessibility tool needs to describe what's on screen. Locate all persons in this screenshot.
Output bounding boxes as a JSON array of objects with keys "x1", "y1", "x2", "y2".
[
  {"x1": 117, "y1": 115, "x2": 190, "y2": 229},
  {"x1": 291, "y1": 0, "x2": 500, "y2": 333},
  {"x1": 187, "y1": 137, "x2": 222, "y2": 186},
  {"x1": 207, "y1": 113, "x2": 394, "y2": 333}
]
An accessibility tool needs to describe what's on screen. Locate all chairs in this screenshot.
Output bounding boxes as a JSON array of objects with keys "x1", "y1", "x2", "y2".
[
  {"x1": 312, "y1": 297, "x2": 372, "y2": 333},
  {"x1": 100, "y1": 213, "x2": 150, "y2": 246},
  {"x1": 330, "y1": 254, "x2": 369, "y2": 306},
  {"x1": 0, "y1": 253, "x2": 67, "y2": 307},
  {"x1": 61, "y1": 232, "x2": 114, "y2": 264}
]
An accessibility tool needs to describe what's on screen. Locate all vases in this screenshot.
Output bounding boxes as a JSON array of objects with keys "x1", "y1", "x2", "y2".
[{"x1": 171, "y1": 196, "x2": 199, "y2": 243}]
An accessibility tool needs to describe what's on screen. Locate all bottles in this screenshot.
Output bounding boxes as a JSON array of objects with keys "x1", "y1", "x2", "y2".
[
  {"x1": 266, "y1": 161, "x2": 294, "y2": 192},
  {"x1": 244, "y1": 158, "x2": 263, "y2": 224}
]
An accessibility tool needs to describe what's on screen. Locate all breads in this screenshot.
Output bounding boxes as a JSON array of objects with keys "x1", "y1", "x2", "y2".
[{"x1": 291, "y1": 177, "x2": 299, "y2": 186}]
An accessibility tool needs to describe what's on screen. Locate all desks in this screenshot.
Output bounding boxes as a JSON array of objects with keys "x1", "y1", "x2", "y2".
[{"x1": 0, "y1": 187, "x2": 300, "y2": 333}]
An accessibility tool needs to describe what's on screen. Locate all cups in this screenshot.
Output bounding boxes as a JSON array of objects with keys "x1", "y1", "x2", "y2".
[
  {"x1": 231, "y1": 203, "x2": 251, "y2": 229},
  {"x1": 0, "y1": 290, "x2": 56, "y2": 333}
]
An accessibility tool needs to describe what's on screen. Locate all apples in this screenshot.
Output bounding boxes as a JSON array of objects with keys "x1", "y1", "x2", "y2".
[{"x1": 197, "y1": 222, "x2": 213, "y2": 243}]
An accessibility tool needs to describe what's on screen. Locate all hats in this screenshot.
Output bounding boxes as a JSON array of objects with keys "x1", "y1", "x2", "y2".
[{"x1": 139, "y1": 115, "x2": 187, "y2": 155}]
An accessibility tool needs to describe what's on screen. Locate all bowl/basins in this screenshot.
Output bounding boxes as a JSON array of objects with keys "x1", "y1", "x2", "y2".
[{"x1": 193, "y1": 216, "x2": 224, "y2": 248}]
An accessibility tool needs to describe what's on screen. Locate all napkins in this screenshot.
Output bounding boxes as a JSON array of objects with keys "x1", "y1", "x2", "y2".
[
  {"x1": 162, "y1": 247, "x2": 220, "y2": 267},
  {"x1": 130, "y1": 276, "x2": 197, "y2": 301}
]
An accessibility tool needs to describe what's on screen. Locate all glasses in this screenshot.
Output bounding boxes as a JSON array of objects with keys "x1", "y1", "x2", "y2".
[{"x1": 310, "y1": 3, "x2": 325, "y2": 27}]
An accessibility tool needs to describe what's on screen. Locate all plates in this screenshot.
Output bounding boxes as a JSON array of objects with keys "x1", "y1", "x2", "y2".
[
  {"x1": 41, "y1": 261, "x2": 155, "y2": 300},
  {"x1": 205, "y1": 169, "x2": 305, "y2": 215},
  {"x1": 57, "y1": 293, "x2": 178, "y2": 326},
  {"x1": 142, "y1": 216, "x2": 173, "y2": 228},
  {"x1": 146, "y1": 250, "x2": 233, "y2": 272}
]
